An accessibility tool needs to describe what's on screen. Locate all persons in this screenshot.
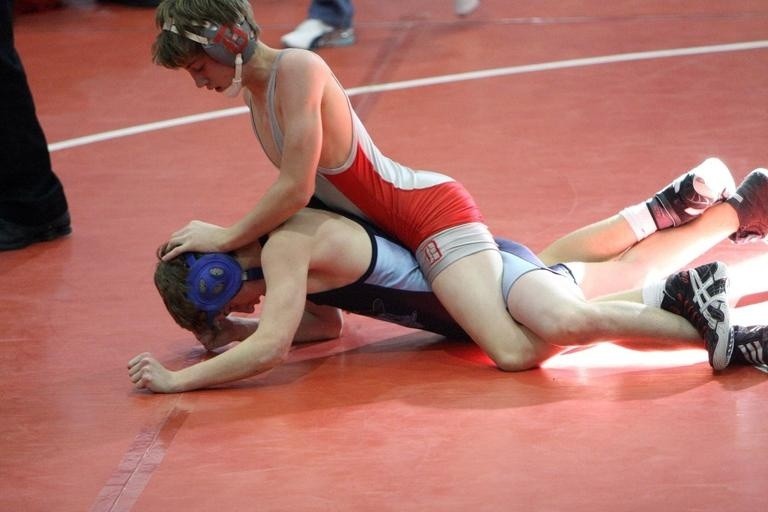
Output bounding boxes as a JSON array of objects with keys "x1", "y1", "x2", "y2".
[
  {"x1": 124, "y1": 157, "x2": 768, "y2": 396},
  {"x1": 151, "y1": 2, "x2": 744, "y2": 379},
  {"x1": 278, "y1": 1, "x2": 355, "y2": 53},
  {"x1": 0, "y1": 2, "x2": 72, "y2": 252}
]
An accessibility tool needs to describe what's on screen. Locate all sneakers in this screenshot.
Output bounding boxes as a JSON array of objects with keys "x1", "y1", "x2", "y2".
[
  {"x1": 658, "y1": 155, "x2": 736, "y2": 226},
  {"x1": 727, "y1": 170, "x2": 768, "y2": 244},
  {"x1": 664, "y1": 261, "x2": 735, "y2": 370},
  {"x1": 279, "y1": 19, "x2": 354, "y2": 49},
  {"x1": 1, "y1": 200, "x2": 73, "y2": 251},
  {"x1": 735, "y1": 321, "x2": 767, "y2": 367}
]
